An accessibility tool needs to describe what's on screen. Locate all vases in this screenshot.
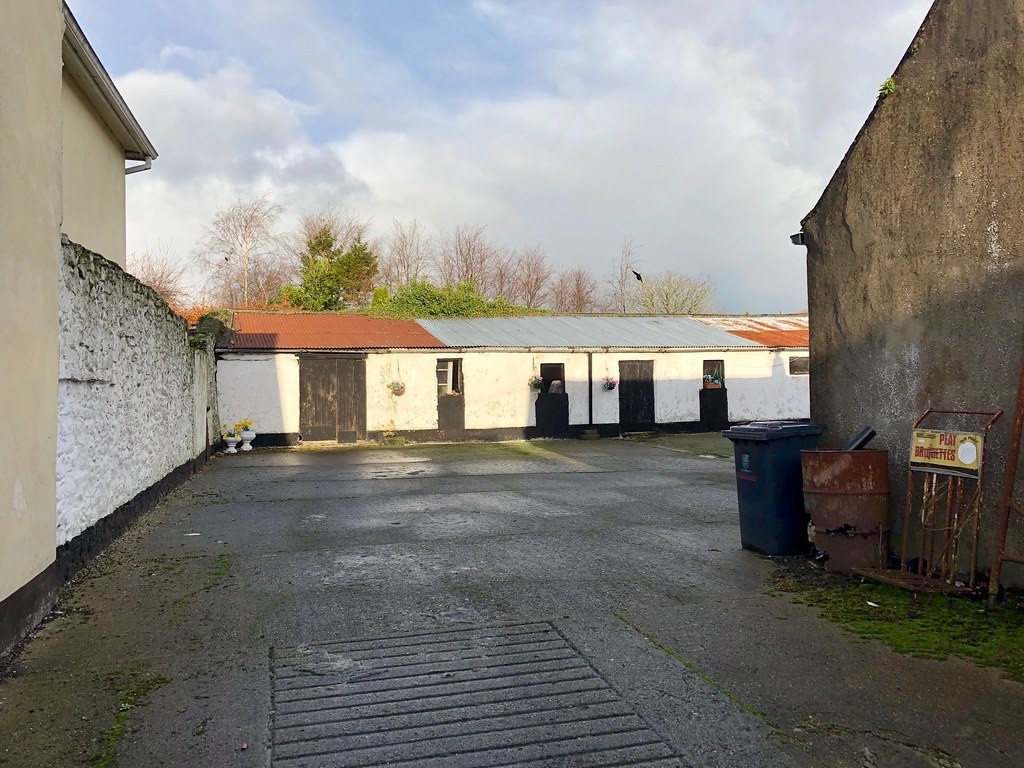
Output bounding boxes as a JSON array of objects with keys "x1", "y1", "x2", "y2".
[
  {"x1": 223, "y1": 437, "x2": 241, "y2": 454},
  {"x1": 534, "y1": 382, "x2": 543, "y2": 389},
  {"x1": 393, "y1": 384, "x2": 406, "y2": 395},
  {"x1": 239, "y1": 431, "x2": 255, "y2": 451},
  {"x1": 607, "y1": 386, "x2": 615, "y2": 390}
]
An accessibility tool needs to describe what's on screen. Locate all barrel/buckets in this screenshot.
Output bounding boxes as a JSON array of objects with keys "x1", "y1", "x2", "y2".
[{"x1": 800, "y1": 447, "x2": 892, "y2": 577}]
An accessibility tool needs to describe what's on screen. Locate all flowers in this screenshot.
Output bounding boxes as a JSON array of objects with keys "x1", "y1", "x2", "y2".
[
  {"x1": 603, "y1": 381, "x2": 616, "y2": 388},
  {"x1": 221, "y1": 424, "x2": 241, "y2": 439},
  {"x1": 235, "y1": 420, "x2": 253, "y2": 431},
  {"x1": 383, "y1": 421, "x2": 400, "y2": 436},
  {"x1": 529, "y1": 375, "x2": 543, "y2": 383},
  {"x1": 447, "y1": 390, "x2": 459, "y2": 397},
  {"x1": 387, "y1": 380, "x2": 404, "y2": 390}
]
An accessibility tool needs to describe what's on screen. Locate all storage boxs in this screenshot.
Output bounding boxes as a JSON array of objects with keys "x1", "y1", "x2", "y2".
[{"x1": 384, "y1": 437, "x2": 405, "y2": 445}]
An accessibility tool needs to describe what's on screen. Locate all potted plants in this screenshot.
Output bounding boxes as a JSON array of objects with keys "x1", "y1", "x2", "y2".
[{"x1": 703, "y1": 375, "x2": 722, "y2": 388}]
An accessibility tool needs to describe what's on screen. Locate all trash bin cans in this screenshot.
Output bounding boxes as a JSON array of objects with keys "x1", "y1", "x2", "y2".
[{"x1": 718, "y1": 417, "x2": 826, "y2": 556}]
